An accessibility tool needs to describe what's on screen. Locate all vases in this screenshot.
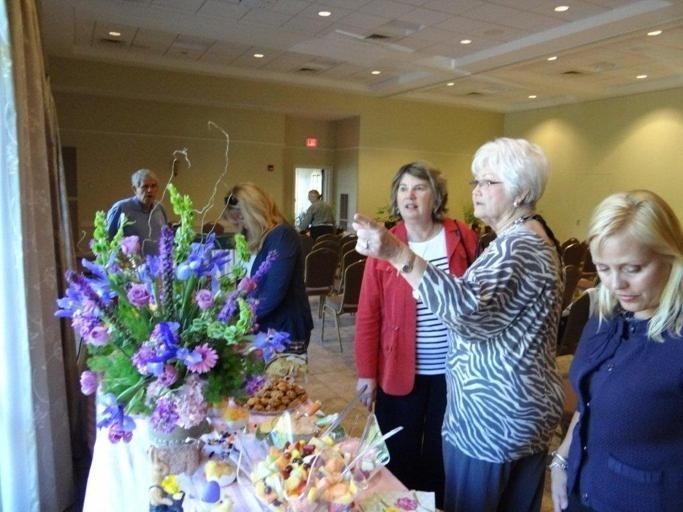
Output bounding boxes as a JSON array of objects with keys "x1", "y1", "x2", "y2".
[{"x1": 143, "y1": 422, "x2": 198, "y2": 477}]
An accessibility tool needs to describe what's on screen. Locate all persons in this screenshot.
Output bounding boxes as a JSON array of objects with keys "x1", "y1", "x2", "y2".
[
  {"x1": 299, "y1": 189, "x2": 336, "y2": 234},
  {"x1": 223, "y1": 184, "x2": 315, "y2": 356},
  {"x1": 352, "y1": 158, "x2": 477, "y2": 509},
  {"x1": 352, "y1": 135, "x2": 568, "y2": 512},
  {"x1": 547, "y1": 188, "x2": 682, "y2": 512},
  {"x1": 104, "y1": 167, "x2": 168, "y2": 256}
]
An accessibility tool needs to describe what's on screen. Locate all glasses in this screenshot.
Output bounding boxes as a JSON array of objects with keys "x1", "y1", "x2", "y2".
[{"x1": 467, "y1": 180, "x2": 506, "y2": 191}]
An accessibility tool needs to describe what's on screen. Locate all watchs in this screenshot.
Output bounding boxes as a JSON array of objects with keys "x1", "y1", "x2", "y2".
[{"x1": 398, "y1": 253, "x2": 416, "y2": 274}]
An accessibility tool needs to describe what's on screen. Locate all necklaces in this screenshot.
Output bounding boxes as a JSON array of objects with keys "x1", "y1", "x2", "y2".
[{"x1": 498, "y1": 214, "x2": 532, "y2": 233}]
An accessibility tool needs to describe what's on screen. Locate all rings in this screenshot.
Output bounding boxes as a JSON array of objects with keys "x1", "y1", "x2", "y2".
[{"x1": 365, "y1": 240, "x2": 370, "y2": 249}]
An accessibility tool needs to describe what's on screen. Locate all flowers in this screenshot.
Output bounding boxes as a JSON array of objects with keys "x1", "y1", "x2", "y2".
[{"x1": 52, "y1": 120, "x2": 292, "y2": 446}]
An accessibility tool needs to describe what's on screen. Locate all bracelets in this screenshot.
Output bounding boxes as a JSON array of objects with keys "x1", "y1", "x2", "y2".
[
  {"x1": 548, "y1": 463, "x2": 568, "y2": 471},
  {"x1": 551, "y1": 451, "x2": 567, "y2": 464}
]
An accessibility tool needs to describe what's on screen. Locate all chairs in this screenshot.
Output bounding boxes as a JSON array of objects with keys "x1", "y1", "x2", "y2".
[{"x1": 194, "y1": 231, "x2": 602, "y2": 358}]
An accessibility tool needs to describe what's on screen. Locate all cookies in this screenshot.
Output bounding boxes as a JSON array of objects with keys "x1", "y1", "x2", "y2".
[{"x1": 245, "y1": 381, "x2": 306, "y2": 411}]
